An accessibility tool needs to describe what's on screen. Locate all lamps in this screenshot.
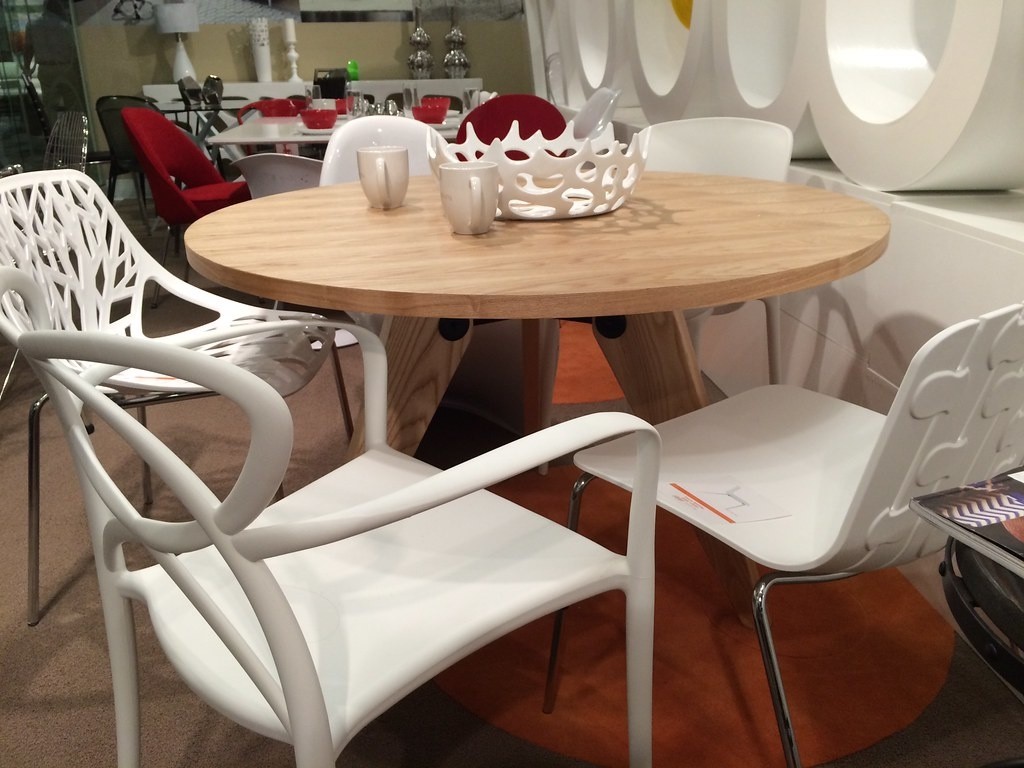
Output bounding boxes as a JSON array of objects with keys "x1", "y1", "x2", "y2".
[{"x1": 153, "y1": 2, "x2": 202, "y2": 83}]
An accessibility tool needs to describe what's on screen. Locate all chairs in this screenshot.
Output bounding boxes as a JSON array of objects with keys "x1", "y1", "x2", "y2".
[
  {"x1": 0, "y1": 111, "x2": 89, "y2": 268},
  {"x1": 17, "y1": 331, "x2": 663, "y2": 768},
  {"x1": 633, "y1": 116, "x2": 794, "y2": 386},
  {"x1": 94, "y1": 87, "x2": 621, "y2": 309},
  {"x1": 543, "y1": 300, "x2": 1024, "y2": 768},
  {"x1": 0, "y1": 169, "x2": 355, "y2": 625}
]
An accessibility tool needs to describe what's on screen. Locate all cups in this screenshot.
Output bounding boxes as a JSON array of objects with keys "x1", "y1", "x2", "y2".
[
  {"x1": 305, "y1": 85, "x2": 323, "y2": 109},
  {"x1": 438, "y1": 160, "x2": 499, "y2": 235},
  {"x1": 356, "y1": 146, "x2": 409, "y2": 209},
  {"x1": 401, "y1": 82, "x2": 418, "y2": 118},
  {"x1": 345, "y1": 90, "x2": 365, "y2": 120},
  {"x1": 384, "y1": 99, "x2": 399, "y2": 115},
  {"x1": 461, "y1": 88, "x2": 481, "y2": 117}
]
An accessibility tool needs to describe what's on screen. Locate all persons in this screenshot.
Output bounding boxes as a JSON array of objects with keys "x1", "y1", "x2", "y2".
[{"x1": 22, "y1": 0, "x2": 86, "y2": 127}]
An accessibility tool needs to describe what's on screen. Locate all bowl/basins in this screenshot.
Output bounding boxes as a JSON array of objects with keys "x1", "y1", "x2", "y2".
[
  {"x1": 421, "y1": 97, "x2": 451, "y2": 111},
  {"x1": 294, "y1": 109, "x2": 338, "y2": 129},
  {"x1": 336, "y1": 98, "x2": 354, "y2": 114},
  {"x1": 411, "y1": 107, "x2": 447, "y2": 124}
]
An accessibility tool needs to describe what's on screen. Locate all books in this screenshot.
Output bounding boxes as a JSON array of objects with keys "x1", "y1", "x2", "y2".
[{"x1": 908, "y1": 464, "x2": 1024, "y2": 579}]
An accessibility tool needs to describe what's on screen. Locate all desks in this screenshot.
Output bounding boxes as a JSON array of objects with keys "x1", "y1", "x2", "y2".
[
  {"x1": 142, "y1": 78, "x2": 483, "y2": 105},
  {"x1": 182, "y1": 170, "x2": 890, "y2": 629},
  {"x1": 205, "y1": 117, "x2": 469, "y2": 154}
]
